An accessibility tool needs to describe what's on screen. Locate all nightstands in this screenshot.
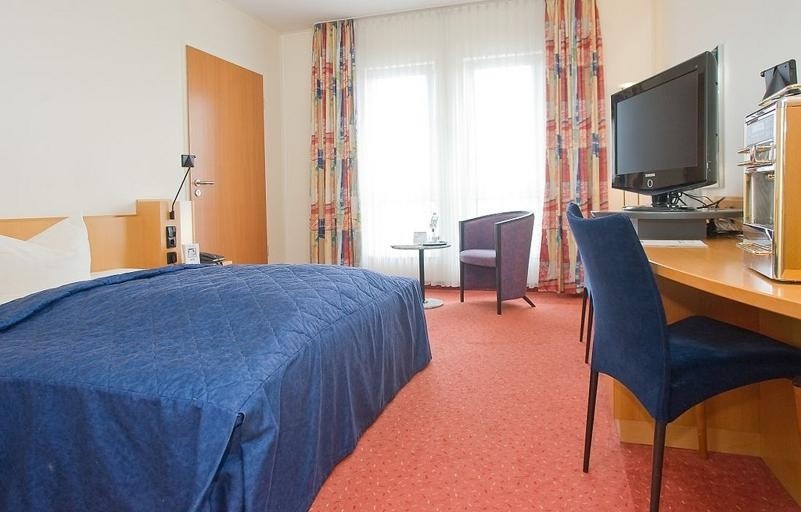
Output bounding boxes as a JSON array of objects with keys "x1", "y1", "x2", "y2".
[{"x1": 201, "y1": 251, "x2": 233, "y2": 265}]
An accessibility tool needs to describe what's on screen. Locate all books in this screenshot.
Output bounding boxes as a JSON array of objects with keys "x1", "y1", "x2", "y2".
[
  {"x1": 640, "y1": 238, "x2": 707, "y2": 250},
  {"x1": 736, "y1": 239, "x2": 771, "y2": 255}
]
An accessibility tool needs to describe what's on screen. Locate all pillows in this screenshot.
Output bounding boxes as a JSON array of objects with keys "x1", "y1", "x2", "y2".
[{"x1": 0, "y1": 217, "x2": 90, "y2": 303}]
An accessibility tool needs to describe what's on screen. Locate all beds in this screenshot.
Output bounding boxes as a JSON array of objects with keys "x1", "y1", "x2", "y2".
[{"x1": 0, "y1": 260, "x2": 432, "y2": 512}]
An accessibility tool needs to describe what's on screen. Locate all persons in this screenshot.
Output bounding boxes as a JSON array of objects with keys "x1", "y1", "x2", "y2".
[{"x1": 188, "y1": 248, "x2": 196, "y2": 258}]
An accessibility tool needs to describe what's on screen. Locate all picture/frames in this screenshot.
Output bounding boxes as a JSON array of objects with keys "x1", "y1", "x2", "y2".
[{"x1": 182, "y1": 243, "x2": 200, "y2": 265}]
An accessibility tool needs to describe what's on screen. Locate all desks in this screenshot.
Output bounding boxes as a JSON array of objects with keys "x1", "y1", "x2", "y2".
[
  {"x1": 612, "y1": 235, "x2": 801, "y2": 510},
  {"x1": 392, "y1": 242, "x2": 452, "y2": 309}
]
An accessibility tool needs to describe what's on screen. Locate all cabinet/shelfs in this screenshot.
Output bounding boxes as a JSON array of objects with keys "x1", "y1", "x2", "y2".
[
  {"x1": 737, "y1": 98, "x2": 800, "y2": 283},
  {"x1": 590, "y1": 207, "x2": 743, "y2": 240}
]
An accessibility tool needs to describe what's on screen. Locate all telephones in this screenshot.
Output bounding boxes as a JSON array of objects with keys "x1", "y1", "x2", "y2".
[{"x1": 200, "y1": 252, "x2": 227, "y2": 264}]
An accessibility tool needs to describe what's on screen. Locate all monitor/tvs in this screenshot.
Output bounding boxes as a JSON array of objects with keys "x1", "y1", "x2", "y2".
[{"x1": 611, "y1": 50, "x2": 719, "y2": 212}]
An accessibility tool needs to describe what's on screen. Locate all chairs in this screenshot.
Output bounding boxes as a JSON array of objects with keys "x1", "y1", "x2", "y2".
[
  {"x1": 566, "y1": 201, "x2": 800, "y2": 512},
  {"x1": 459, "y1": 210, "x2": 537, "y2": 315}
]
